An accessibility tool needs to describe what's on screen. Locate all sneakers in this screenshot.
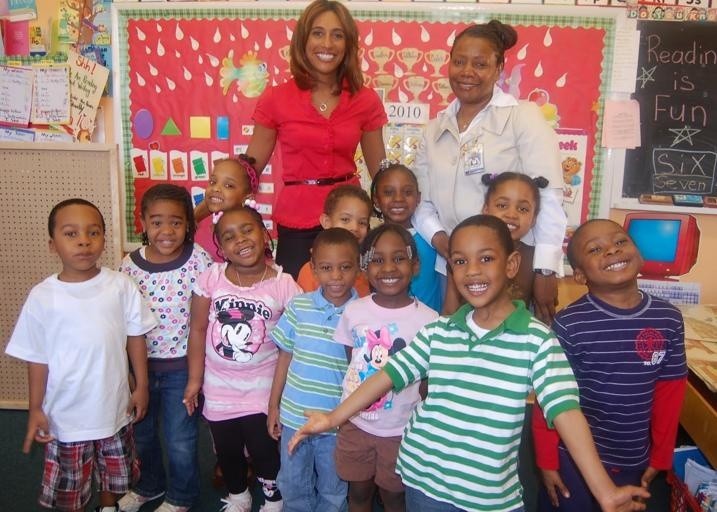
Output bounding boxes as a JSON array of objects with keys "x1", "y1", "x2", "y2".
[
  {"x1": 222, "y1": 492, "x2": 253, "y2": 512},
  {"x1": 258, "y1": 497, "x2": 283, "y2": 512},
  {"x1": 115, "y1": 487, "x2": 165, "y2": 512},
  {"x1": 152, "y1": 497, "x2": 192, "y2": 512}
]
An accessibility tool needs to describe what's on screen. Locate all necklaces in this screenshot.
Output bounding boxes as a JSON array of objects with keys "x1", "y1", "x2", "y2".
[
  {"x1": 459, "y1": 104, "x2": 481, "y2": 131},
  {"x1": 311, "y1": 87, "x2": 335, "y2": 112}
]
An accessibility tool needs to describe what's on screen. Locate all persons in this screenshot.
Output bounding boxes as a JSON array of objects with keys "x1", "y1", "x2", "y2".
[
  {"x1": 410, "y1": 19, "x2": 568, "y2": 326},
  {"x1": 287, "y1": 213, "x2": 651, "y2": 512},
  {"x1": 439, "y1": 171, "x2": 550, "y2": 512},
  {"x1": 533, "y1": 218, "x2": 689, "y2": 511},
  {"x1": 195, "y1": 0, "x2": 388, "y2": 286}
]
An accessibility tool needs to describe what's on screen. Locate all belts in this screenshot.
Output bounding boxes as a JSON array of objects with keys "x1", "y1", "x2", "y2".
[{"x1": 283, "y1": 172, "x2": 355, "y2": 187}]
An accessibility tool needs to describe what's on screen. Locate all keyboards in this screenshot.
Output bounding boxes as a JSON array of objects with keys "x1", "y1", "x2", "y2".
[{"x1": 637, "y1": 279, "x2": 701, "y2": 305}]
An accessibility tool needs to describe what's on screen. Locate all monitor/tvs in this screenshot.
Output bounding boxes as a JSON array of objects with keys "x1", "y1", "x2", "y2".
[{"x1": 624, "y1": 211, "x2": 702, "y2": 281}]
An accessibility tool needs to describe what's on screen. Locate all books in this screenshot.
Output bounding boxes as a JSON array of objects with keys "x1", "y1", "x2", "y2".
[{"x1": 671, "y1": 441, "x2": 717, "y2": 512}]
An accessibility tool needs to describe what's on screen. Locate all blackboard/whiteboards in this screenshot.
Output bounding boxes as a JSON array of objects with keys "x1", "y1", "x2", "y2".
[{"x1": 608, "y1": 5, "x2": 717, "y2": 215}]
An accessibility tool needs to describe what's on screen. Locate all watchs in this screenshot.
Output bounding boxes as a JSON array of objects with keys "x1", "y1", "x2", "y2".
[{"x1": 535, "y1": 269, "x2": 555, "y2": 277}]
